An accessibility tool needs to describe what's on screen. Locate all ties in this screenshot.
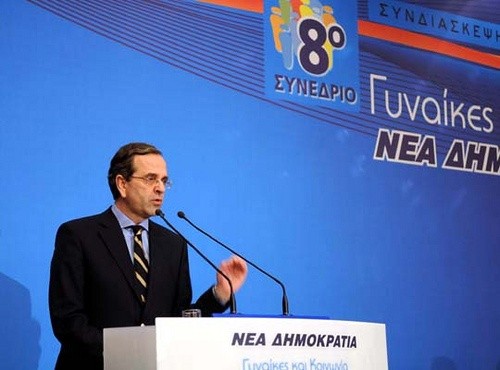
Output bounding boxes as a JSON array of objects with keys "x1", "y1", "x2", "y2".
[{"x1": 131, "y1": 226, "x2": 149, "y2": 304}]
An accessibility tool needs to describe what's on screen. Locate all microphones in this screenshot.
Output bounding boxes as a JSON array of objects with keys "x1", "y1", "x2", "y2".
[
  {"x1": 177, "y1": 211, "x2": 289, "y2": 314},
  {"x1": 155, "y1": 209, "x2": 236, "y2": 314}
]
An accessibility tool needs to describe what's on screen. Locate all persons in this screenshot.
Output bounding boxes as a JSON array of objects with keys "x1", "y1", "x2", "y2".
[{"x1": 49, "y1": 142, "x2": 247, "y2": 370}]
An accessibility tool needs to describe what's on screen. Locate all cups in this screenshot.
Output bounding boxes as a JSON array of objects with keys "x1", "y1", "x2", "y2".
[{"x1": 178, "y1": 309, "x2": 201, "y2": 317}]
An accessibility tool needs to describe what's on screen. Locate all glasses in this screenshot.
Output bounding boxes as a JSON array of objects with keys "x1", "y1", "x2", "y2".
[{"x1": 131, "y1": 173, "x2": 172, "y2": 189}]
